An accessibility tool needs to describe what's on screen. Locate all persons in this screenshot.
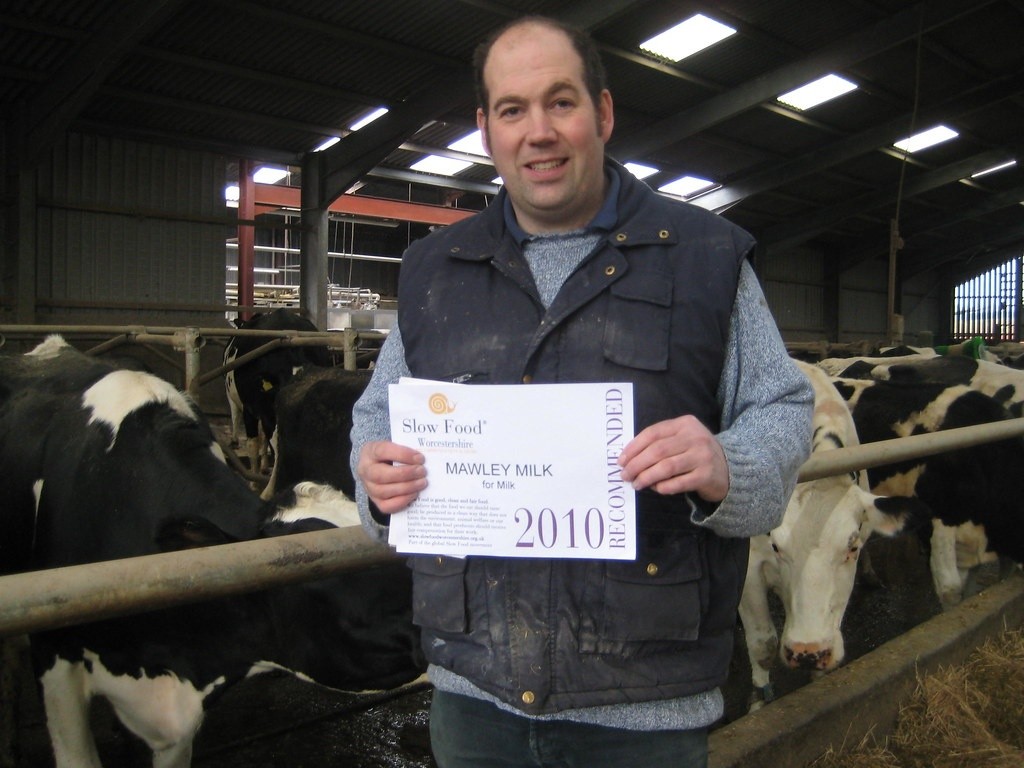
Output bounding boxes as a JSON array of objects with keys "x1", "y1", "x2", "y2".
[{"x1": 349, "y1": 16, "x2": 815, "y2": 768}]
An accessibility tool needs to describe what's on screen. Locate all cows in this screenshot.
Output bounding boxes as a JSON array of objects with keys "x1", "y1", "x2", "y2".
[
  {"x1": 731, "y1": 341, "x2": 1024, "y2": 717},
  {"x1": 0, "y1": 307, "x2": 428, "y2": 768}
]
